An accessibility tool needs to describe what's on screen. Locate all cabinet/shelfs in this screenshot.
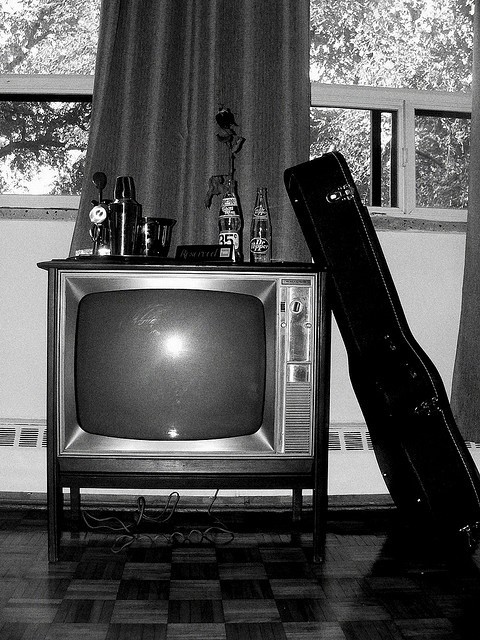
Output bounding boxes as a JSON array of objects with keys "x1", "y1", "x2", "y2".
[{"x1": 38, "y1": 257, "x2": 333, "y2": 561}]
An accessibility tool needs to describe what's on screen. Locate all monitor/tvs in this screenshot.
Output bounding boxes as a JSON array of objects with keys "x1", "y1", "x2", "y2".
[{"x1": 56, "y1": 268, "x2": 316, "y2": 461}]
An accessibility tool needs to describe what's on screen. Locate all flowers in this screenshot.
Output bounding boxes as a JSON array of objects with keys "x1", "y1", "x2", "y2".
[{"x1": 204, "y1": 106, "x2": 244, "y2": 207}]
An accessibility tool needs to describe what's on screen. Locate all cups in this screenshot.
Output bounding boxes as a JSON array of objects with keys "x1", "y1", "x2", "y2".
[{"x1": 136, "y1": 217, "x2": 177, "y2": 257}]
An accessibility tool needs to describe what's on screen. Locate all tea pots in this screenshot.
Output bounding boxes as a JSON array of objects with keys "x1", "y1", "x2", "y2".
[{"x1": 89, "y1": 176, "x2": 143, "y2": 257}]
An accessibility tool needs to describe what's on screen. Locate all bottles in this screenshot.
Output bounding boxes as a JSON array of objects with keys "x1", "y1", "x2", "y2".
[
  {"x1": 218, "y1": 180, "x2": 244, "y2": 262},
  {"x1": 250, "y1": 187, "x2": 272, "y2": 263}
]
